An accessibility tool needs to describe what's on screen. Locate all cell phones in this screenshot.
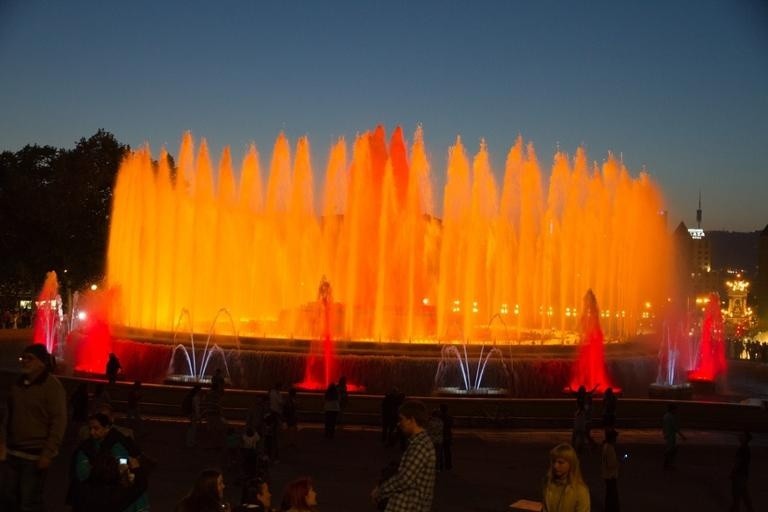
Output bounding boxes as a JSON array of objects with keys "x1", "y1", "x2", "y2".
[{"x1": 119, "y1": 457, "x2": 128, "y2": 465}]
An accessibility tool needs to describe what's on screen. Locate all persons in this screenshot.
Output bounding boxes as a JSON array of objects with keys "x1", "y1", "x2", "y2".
[
  {"x1": 662, "y1": 405, "x2": 685, "y2": 468},
  {"x1": 571, "y1": 387, "x2": 618, "y2": 456},
  {"x1": 1, "y1": 345, "x2": 452, "y2": 511},
  {"x1": 601, "y1": 428, "x2": 619, "y2": 510},
  {"x1": 726, "y1": 338, "x2": 768, "y2": 361},
  {"x1": 0, "y1": 303, "x2": 33, "y2": 329},
  {"x1": 543, "y1": 443, "x2": 592, "y2": 511},
  {"x1": 729, "y1": 430, "x2": 754, "y2": 512}
]
